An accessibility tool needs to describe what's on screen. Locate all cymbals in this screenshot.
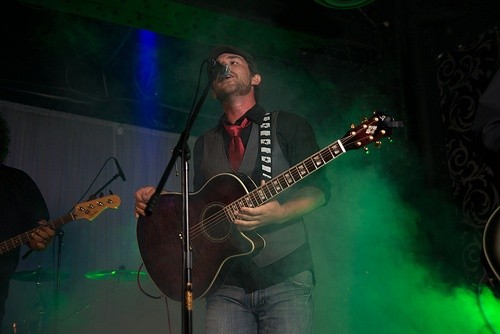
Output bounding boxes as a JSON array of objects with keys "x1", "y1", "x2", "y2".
[
  {"x1": 84, "y1": 268, "x2": 149, "y2": 281},
  {"x1": 11, "y1": 270, "x2": 74, "y2": 284}
]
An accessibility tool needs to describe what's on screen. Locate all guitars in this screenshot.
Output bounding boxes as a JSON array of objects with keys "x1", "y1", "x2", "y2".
[
  {"x1": 1, "y1": 190, "x2": 122, "y2": 259},
  {"x1": 479, "y1": 204, "x2": 500, "y2": 299},
  {"x1": 135, "y1": 112, "x2": 398, "y2": 303}
]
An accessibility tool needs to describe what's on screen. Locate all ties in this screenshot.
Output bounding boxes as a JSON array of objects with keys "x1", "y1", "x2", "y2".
[{"x1": 223, "y1": 118, "x2": 251, "y2": 171}]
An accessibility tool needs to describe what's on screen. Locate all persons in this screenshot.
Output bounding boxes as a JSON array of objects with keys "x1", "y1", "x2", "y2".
[
  {"x1": 134, "y1": 47, "x2": 332, "y2": 334},
  {"x1": 0, "y1": 117, "x2": 56, "y2": 334}
]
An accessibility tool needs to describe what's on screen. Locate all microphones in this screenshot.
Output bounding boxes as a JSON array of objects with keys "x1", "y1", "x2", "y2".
[
  {"x1": 112, "y1": 157, "x2": 126, "y2": 181},
  {"x1": 206, "y1": 58, "x2": 229, "y2": 75}
]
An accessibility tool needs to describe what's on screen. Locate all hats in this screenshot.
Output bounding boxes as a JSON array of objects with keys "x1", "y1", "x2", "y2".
[{"x1": 207, "y1": 46, "x2": 260, "y2": 104}]
